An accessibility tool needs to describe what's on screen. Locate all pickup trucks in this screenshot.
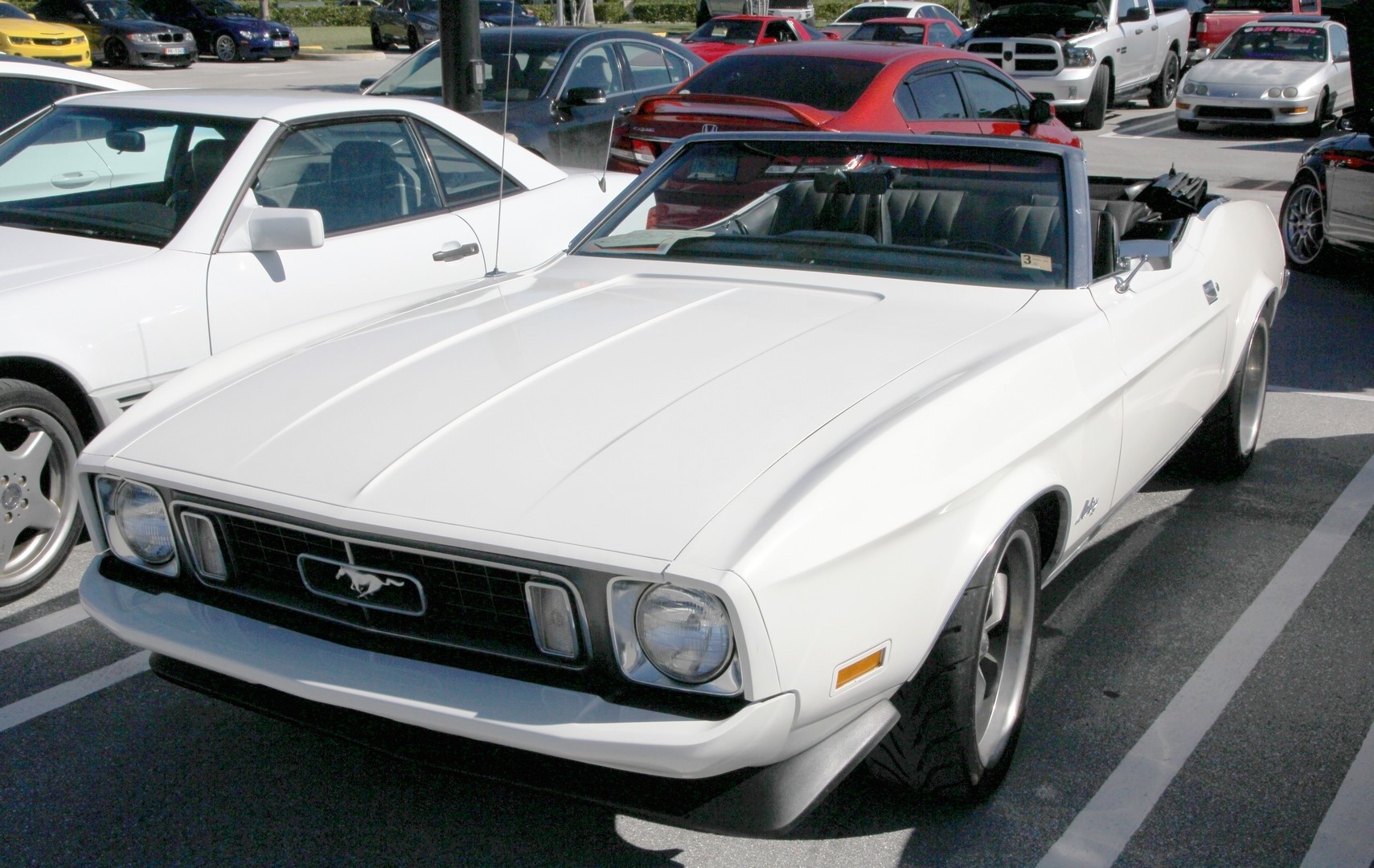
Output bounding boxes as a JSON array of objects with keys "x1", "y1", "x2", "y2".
[
  {"x1": 950, "y1": 0, "x2": 1194, "y2": 131},
  {"x1": 1196, "y1": 0, "x2": 1321, "y2": 51}
]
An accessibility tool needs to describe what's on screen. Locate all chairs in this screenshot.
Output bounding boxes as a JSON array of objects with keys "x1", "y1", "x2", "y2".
[
  {"x1": 484, "y1": 56, "x2": 536, "y2": 102},
  {"x1": 581, "y1": 55, "x2": 613, "y2": 95},
  {"x1": 772, "y1": 175, "x2": 1149, "y2": 278},
  {"x1": 1253, "y1": 35, "x2": 1274, "y2": 53},
  {"x1": 304, "y1": 140, "x2": 421, "y2": 236},
  {"x1": 166, "y1": 138, "x2": 280, "y2": 208}
]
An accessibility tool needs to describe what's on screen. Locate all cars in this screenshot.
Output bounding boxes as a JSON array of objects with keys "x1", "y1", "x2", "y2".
[
  {"x1": 767, "y1": 0, "x2": 817, "y2": 29},
  {"x1": 1152, "y1": 0, "x2": 1207, "y2": 39},
  {"x1": 369, "y1": 1, "x2": 545, "y2": 54},
  {"x1": 1276, "y1": 132, "x2": 1374, "y2": 273},
  {"x1": 26, "y1": 0, "x2": 198, "y2": 70},
  {"x1": 825, "y1": 0, "x2": 973, "y2": 40},
  {"x1": 74, "y1": 128, "x2": 1295, "y2": 817},
  {"x1": 605, "y1": 39, "x2": 1085, "y2": 230},
  {"x1": 347, "y1": 26, "x2": 711, "y2": 171},
  {"x1": 335, "y1": 0, "x2": 380, "y2": 7},
  {"x1": 627, "y1": 14, "x2": 842, "y2": 68},
  {"x1": 0, "y1": 85, "x2": 660, "y2": 603},
  {"x1": 1174, "y1": 14, "x2": 1355, "y2": 140},
  {"x1": 842, "y1": 17, "x2": 961, "y2": 48},
  {"x1": 0, "y1": 0, "x2": 93, "y2": 70},
  {"x1": 0, "y1": 55, "x2": 224, "y2": 205},
  {"x1": 130, "y1": 0, "x2": 300, "y2": 64}
]
